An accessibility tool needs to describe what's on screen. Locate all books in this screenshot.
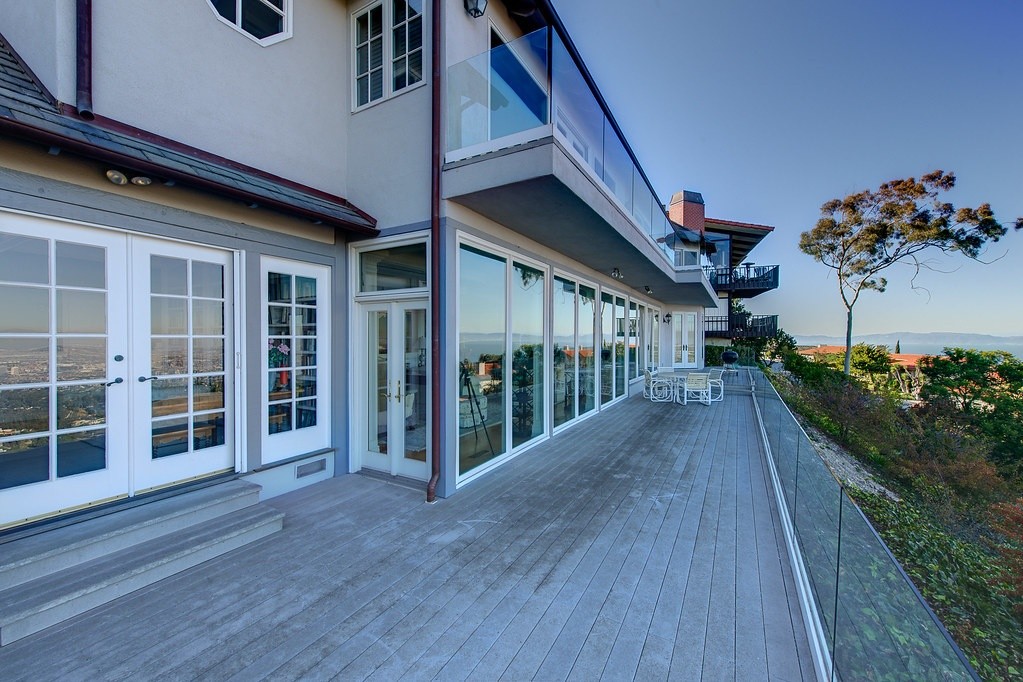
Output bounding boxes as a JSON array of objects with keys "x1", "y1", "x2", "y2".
[{"x1": 269, "y1": 301, "x2": 317, "y2": 429}]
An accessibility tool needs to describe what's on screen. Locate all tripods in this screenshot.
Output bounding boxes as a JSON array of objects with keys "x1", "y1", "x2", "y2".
[{"x1": 458, "y1": 368, "x2": 495, "y2": 456}]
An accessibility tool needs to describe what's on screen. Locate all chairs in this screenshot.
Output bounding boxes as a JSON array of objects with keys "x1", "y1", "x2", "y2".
[{"x1": 643, "y1": 366, "x2": 726, "y2": 406}]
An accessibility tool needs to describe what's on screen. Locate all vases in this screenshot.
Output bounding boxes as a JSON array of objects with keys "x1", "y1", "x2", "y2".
[{"x1": 269, "y1": 364, "x2": 277, "y2": 392}]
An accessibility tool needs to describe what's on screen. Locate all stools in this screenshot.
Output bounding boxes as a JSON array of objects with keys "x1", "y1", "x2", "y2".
[
  {"x1": 269, "y1": 413, "x2": 289, "y2": 432},
  {"x1": 152, "y1": 423, "x2": 219, "y2": 457}
]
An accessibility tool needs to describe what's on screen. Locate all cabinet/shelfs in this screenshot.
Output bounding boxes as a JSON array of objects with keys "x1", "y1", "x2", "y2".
[{"x1": 268, "y1": 297, "x2": 316, "y2": 411}]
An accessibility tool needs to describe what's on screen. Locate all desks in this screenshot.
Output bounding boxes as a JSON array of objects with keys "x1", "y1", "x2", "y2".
[
  {"x1": 152, "y1": 388, "x2": 305, "y2": 417},
  {"x1": 658, "y1": 372, "x2": 688, "y2": 403}
]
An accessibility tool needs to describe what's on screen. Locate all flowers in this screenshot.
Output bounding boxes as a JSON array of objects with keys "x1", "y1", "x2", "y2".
[{"x1": 268, "y1": 338, "x2": 290, "y2": 365}]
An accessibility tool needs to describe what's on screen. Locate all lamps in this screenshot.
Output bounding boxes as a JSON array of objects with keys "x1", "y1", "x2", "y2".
[
  {"x1": 106, "y1": 165, "x2": 153, "y2": 187},
  {"x1": 663, "y1": 312, "x2": 672, "y2": 325},
  {"x1": 655, "y1": 314, "x2": 658, "y2": 322},
  {"x1": 645, "y1": 286, "x2": 653, "y2": 294},
  {"x1": 611, "y1": 268, "x2": 624, "y2": 279},
  {"x1": 418, "y1": 336, "x2": 426, "y2": 367},
  {"x1": 464, "y1": 0, "x2": 488, "y2": 19}
]
